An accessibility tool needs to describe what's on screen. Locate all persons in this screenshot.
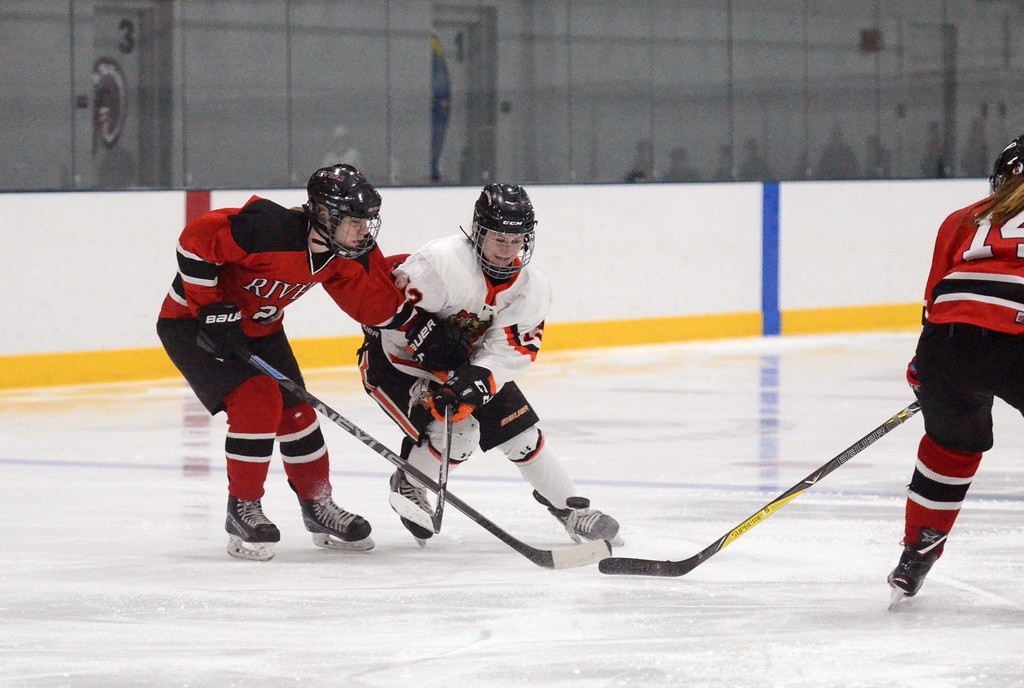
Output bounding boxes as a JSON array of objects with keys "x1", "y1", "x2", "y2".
[
  {"x1": 359, "y1": 184, "x2": 627, "y2": 548},
  {"x1": 626, "y1": 99, "x2": 1009, "y2": 183},
  {"x1": 887, "y1": 133, "x2": 1024, "y2": 607},
  {"x1": 157, "y1": 164, "x2": 443, "y2": 561}
]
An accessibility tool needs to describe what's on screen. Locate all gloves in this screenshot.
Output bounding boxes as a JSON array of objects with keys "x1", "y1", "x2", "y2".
[
  {"x1": 408, "y1": 307, "x2": 464, "y2": 371},
  {"x1": 200, "y1": 304, "x2": 248, "y2": 358},
  {"x1": 906, "y1": 355, "x2": 923, "y2": 395},
  {"x1": 429, "y1": 366, "x2": 494, "y2": 422}
]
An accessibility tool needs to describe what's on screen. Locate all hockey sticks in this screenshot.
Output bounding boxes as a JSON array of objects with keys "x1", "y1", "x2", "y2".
[
  {"x1": 388, "y1": 402, "x2": 453, "y2": 535},
  {"x1": 597, "y1": 400, "x2": 920, "y2": 577},
  {"x1": 247, "y1": 353, "x2": 612, "y2": 572}
]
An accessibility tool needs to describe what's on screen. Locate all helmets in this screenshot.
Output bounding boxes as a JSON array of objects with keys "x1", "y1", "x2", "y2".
[
  {"x1": 991, "y1": 135, "x2": 1024, "y2": 176},
  {"x1": 472, "y1": 183, "x2": 537, "y2": 279},
  {"x1": 307, "y1": 162, "x2": 382, "y2": 259}
]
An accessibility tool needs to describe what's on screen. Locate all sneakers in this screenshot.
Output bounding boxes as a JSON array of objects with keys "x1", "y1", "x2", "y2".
[
  {"x1": 887, "y1": 526, "x2": 947, "y2": 612},
  {"x1": 389, "y1": 436, "x2": 436, "y2": 546},
  {"x1": 223, "y1": 494, "x2": 281, "y2": 561},
  {"x1": 286, "y1": 478, "x2": 375, "y2": 551},
  {"x1": 535, "y1": 491, "x2": 622, "y2": 546}
]
